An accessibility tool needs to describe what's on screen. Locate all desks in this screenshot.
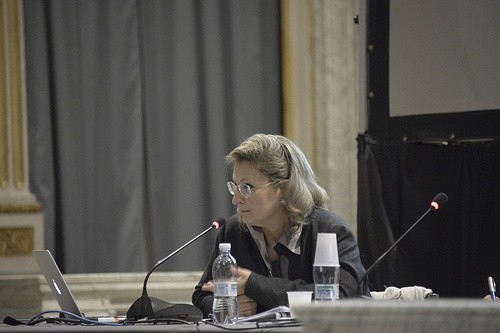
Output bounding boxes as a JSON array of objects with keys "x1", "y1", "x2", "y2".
[{"x1": 0, "y1": 300, "x2": 500, "y2": 333}]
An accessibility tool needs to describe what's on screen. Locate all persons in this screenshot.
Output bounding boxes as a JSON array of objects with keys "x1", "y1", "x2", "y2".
[{"x1": 192, "y1": 134, "x2": 372, "y2": 320}]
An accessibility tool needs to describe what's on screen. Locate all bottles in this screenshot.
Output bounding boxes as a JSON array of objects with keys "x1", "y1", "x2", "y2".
[
  {"x1": 312, "y1": 266, "x2": 340, "y2": 301},
  {"x1": 212, "y1": 243, "x2": 239, "y2": 325}
]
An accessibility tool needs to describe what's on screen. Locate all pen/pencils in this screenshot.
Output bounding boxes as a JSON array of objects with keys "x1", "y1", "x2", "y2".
[{"x1": 487, "y1": 276, "x2": 496, "y2": 300}]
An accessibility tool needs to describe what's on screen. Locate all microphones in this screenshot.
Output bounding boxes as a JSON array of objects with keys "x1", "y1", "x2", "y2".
[
  {"x1": 357, "y1": 193, "x2": 448, "y2": 294},
  {"x1": 127, "y1": 217, "x2": 226, "y2": 323}
]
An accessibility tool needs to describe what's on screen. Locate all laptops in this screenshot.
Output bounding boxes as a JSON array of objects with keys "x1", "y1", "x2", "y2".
[{"x1": 32, "y1": 249, "x2": 127, "y2": 323}]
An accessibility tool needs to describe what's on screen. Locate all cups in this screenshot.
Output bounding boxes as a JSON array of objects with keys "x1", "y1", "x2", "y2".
[
  {"x1": 286, "y1": 290, "x2": 313, "y2": 317},
  {"x1": 312, "y1": 232, "x2": 341, "y2": 267}
]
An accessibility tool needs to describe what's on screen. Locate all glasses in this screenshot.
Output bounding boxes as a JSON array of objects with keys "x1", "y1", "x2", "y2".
[{"x1": 227, "y1": 178, "x2": 288, "y2": 198}]
